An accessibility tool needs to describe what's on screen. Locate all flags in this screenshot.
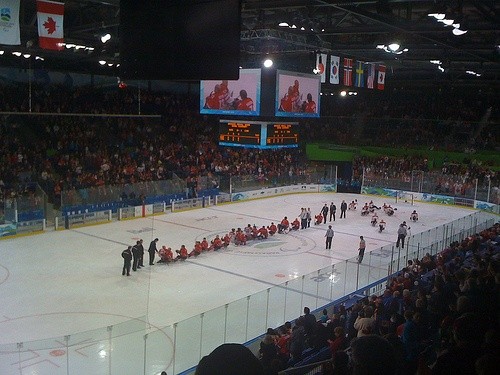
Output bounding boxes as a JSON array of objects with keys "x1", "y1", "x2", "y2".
[
  {"x1": 377, "y1": 65, "x2": 387, "y2": 91},
  {"x1": 344, "y1": 58, "x2": 352, "y2": 86},
  {"x1": 37, "y1": 1, "x2": 64, "y2": 51},
  {"x1": 0, "y1": 0, "x2": 20, "y2": 45},
  {"x1": 354, "y1": 61, "x2": 364, "y2": 87},
  {"x1": 366, "y1": 63, "x2": 376, "y2": 89},
  {"x1": 316, "y1": 54, "x2": 327, "y2": 83},
  {"x1": 329, "y1": 56, "x2": 340, "y2": 85}
]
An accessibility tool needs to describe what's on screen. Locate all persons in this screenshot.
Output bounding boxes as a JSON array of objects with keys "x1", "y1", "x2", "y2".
[
  {"x1": 147, "y1": 237, "x2": 159, "y2": 266},
  {"x1": 122, "y1": 238, "x2": 145, "y2": 276},
  {"x1": 279, "y1": 79, "x2": 317, "y2": 113},
  {"x1": 159, "y1": 222, "x2": 276, "y2": 264},
  {"x1": 194, "y1": 220, "x2": 500, "y2": 375},
  {"x1": 203, "y1": 78, "x2": 253, "y2": 110},
  {"x1": 276, "y1": 198, "x2": 396, "y2": 234},
  {"x1": 409, "y1": 209, "x2": 418, "y2": 223},
  {"x1": 0, "y1": 86, "x2": 500, "y2": 211}
]
